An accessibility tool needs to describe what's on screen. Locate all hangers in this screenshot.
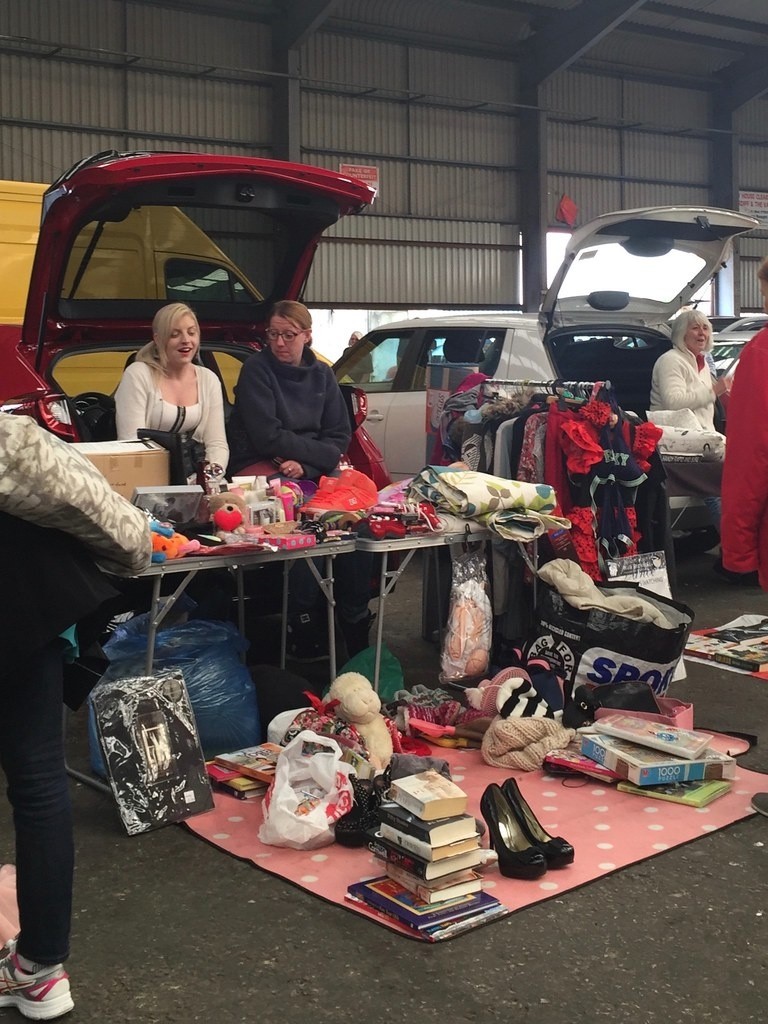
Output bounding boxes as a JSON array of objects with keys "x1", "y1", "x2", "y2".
[{"x1": 477, "y1": 377, "x2": 603, "y2": 415}]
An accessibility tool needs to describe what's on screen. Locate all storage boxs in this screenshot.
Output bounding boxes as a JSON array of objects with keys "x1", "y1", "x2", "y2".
[
  {"x1": 580, "y1": 734, "x2": 736, "y2": 786},
  {"x1": 66, "y1": 436, "x2": 170, "y2": 503}
]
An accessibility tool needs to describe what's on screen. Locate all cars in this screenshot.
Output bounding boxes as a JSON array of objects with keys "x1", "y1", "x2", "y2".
[{"x1": 617, "y1": 314, "x2": 768, "y2": 383}]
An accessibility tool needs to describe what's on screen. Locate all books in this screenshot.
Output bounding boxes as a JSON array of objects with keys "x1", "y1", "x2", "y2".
[
  {"x1": 682, "y1": 624, "x2": 768, "y2": 672},
  {"x1": 546, "y1": 712, "x2": 737, "y2": 810},
  {"x1": 207, "y1": 739, "x2": 286, "y2": 801},
  {"x1": 342, "y1": 765, "x2": 509, "y2": 941}
]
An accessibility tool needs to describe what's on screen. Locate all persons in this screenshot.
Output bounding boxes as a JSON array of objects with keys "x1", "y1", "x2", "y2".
[
  {"x1": 0, "y1": 415, "x2": 127, "y2": 1021},
  {"x1": 718, "y1": 258, "x2": 768, "y2": 815},
  {"x1": 225, "y1": 298, "x2": 352, "y2": 488},
  {"x1": 648, "y1": 311, "x2": 733, "y2": 430},
  {"x1": 343, "y1": 331, "x2": 373, "y2": 383},
  {"x1": 112, "y1": 303, "x2": 231, "y2": 496}
]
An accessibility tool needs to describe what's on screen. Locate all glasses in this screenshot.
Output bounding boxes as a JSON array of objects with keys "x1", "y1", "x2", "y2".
[{"x1": 264, "y1": 327, "x2": 303, "y2": 343}]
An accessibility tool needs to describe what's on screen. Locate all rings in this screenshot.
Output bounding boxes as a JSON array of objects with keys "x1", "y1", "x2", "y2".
[{"x1": 287, "y1": 467, "x2": 292, "y2": 473}]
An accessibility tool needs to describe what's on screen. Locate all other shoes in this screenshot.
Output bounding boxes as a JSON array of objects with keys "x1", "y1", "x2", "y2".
[
  {"x1": 396, "y1": 701, "x2": 459, "y2": 738},
  {"x1": 394, "y1": 684, "x2": 453, "y2": 707},
  {"x1": 298, "y1": 469, "x2": 379, "y2": 513},
  {"x1": 750, "y1": 792, "x2": 768, "y2": 817}
]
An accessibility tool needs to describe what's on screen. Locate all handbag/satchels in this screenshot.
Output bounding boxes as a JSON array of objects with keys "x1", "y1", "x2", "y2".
[
  {"x1": 258, "y1": 731, "x2": 357, "y2": 851},
  {"x1": 521, "y1": 581, "x2": 706, "y2": 729},
  {"x1": 323, "y1": 640, "x2": 405, "y2": 705},
  {"x1": 440, "y1": 555, "x2": 493, "y2": 683},
  {"x1": 280, "y1": 710, "x2": 372, "y2": 759},
  {"x1": 383, "y1": 755, "x2": 453, "y2": 787}
]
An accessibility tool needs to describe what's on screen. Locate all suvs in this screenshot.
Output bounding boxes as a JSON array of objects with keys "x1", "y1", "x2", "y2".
[
  {"x1": 328, "y1": 206, "x2": 759, "y2": 489},
  {"x1": 0, "y1": 150, "x2": 392, "y2": 493}
]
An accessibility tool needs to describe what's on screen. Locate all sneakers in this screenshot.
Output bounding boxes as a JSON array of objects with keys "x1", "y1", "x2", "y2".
[{"x1": 0, "y1": 951, "x2": 74, "y2": 1020}]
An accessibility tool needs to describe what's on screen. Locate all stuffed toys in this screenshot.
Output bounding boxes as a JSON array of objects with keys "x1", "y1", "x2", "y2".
[
  {"x1": 322, "y1": 673, "x2": 392, "y2": 779},
  {"x1": 149, "y1": 521, "x2": 202, "y2": 564},
  {"x1": 207, "y1": 493, "x2": 249, "y2": 539}
]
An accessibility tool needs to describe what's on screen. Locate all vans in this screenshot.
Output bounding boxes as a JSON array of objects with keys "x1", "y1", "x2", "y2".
[{"x1": 0, "y1": 180, "x2": 357, "y2": 403}]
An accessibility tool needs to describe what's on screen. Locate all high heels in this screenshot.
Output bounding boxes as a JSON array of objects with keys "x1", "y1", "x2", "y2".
[
  {"x1": 335, "y1": 774, "x2": 389, "y2": 848},
  {"x1": 480, "y1": 777, "x2": 574, "y2": 879}
]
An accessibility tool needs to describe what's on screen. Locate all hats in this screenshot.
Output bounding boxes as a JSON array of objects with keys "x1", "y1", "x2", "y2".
[{"x1": 463, "y1": 668, "x2": 556, "y2": 719}]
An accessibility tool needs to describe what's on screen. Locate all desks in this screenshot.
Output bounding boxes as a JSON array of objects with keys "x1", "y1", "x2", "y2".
[
  {"x1": 344, "y1": 527, "x2": 540, "y2": 700},
  {"x1": 60, "y1": 538, "x2": 357, "y2": 796}
]
